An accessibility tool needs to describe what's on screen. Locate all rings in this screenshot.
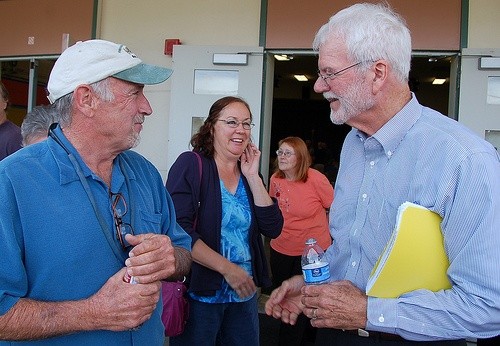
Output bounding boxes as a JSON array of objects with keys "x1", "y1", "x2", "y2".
[{"x1": 312, "y1": 309, "x2": 318, "y2": 320}]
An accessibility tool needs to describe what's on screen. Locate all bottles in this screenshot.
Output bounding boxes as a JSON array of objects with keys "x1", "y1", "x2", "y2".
[{"x1": 301, "y1": 237, "x2": 330, "y2": 286}]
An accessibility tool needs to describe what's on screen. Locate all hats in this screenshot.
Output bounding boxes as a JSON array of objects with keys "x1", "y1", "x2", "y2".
[{"x1": 46, "y1": 39, "x2": 172, "y2": 105}]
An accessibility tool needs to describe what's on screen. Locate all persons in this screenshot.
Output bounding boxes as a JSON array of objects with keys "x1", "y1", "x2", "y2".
[
  {"x1": 166, "y1": 96, "x2": 284, "y2": 346},
  {"x1": 0, "y1": 83, "x2": 22, "y2": 161},
  {"x1": 268, "y1": 137, "x2": 335, "y2": 346},
  {"x1": 21, "y1": 105, "x2": 60, "y2": 148},
  {"x1": 0, "y1": 39, "x2": 191, "y2": 346},
  {"x1": 264, "y1": 3, "x2": 500, "y2": 346}
]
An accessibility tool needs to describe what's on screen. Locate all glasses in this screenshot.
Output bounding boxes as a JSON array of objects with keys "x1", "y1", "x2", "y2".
[
  {"x1": 108, "y1": 192, "x2": 136, "y2": 250},
  {"x1": 215, "y1": 115, "x2": 255, "y2": 131},
  {"x1": 276, "y1": 149, "x2": 297, "y2": 158},
  {"x1": 316, "y1": 57, "x2": 379, "y2": 86}
]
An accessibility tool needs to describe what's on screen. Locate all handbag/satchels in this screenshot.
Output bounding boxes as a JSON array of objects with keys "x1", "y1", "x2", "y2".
[{"x1": 159, "y1": 281, "x2": 188, "y2": 335}]
